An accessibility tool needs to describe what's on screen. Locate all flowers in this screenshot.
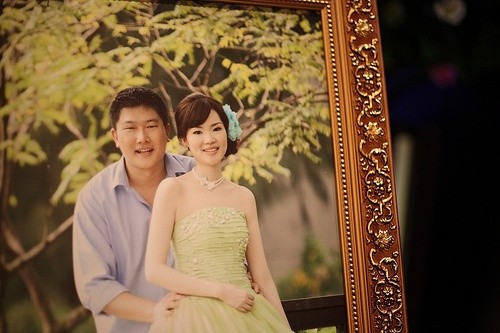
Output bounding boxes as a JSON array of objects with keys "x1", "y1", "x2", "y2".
[{"x1": 222, "y1": 103, "x2": 242, "y2": 141}]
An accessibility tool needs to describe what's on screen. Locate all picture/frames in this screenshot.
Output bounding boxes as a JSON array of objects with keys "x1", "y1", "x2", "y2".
[{"x1": 0, "y1": 0, "x2": 409, "y2": 333}]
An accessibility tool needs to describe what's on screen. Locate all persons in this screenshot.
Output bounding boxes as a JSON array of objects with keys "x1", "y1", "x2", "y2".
[
  {"x1": 145, "y1": 93, "x2": 296, "y2": 333},
  {"x1": 72, "y1": 88, "x2": 198, "y2": 333}
]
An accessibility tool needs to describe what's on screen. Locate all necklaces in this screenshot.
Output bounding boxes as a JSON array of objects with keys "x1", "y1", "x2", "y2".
[{"x1": 192, "y1": 168, "x2": 225, "y2": 192}]
